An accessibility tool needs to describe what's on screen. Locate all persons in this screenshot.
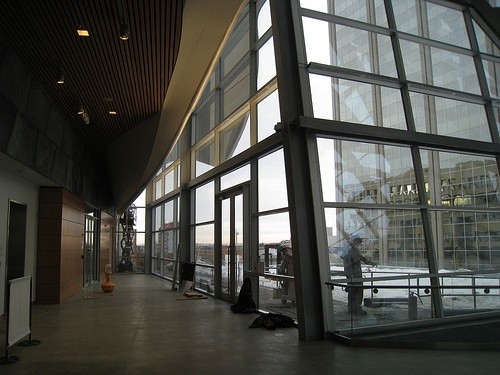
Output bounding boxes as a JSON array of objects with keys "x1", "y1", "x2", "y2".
[{"x1": 343, "y1": 237, "x2": 378, "y2": 315}]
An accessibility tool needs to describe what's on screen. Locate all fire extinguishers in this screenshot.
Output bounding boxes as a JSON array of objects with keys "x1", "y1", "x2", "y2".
[{"x1": 408, "y1": 292, "x2": 423, "y2": 320}]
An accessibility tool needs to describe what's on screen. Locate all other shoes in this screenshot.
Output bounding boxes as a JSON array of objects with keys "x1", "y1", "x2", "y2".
[{"x1": 349, "y1": 309, "x2": 367, "y2": 315}]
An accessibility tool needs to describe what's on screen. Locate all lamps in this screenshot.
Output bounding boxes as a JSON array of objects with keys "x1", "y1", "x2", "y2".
[
  {"x1": 57, "y1": 64, "x2": 64, "y2": 83},
  {"x1": 119, "y1": 25, "x2": 130, "y2": 40},
  {"x1": 77, "y1": 106, "x2": 90, "y2": 124}
]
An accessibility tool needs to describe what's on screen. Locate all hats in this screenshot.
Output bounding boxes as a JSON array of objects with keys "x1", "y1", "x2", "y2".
[{"x1": 351, "y1": 235, "x2": 362, "y2": 243}]
